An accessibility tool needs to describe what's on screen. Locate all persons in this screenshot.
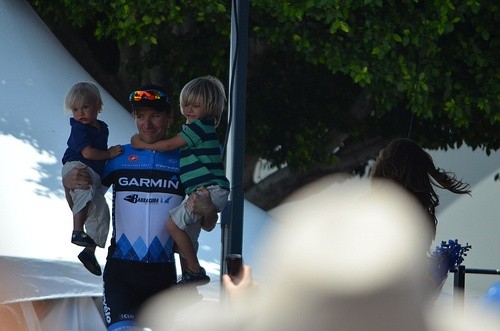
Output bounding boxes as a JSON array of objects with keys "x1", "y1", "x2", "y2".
[
  {"x1": 62, "y1": 89, "x2": 218, "y2": 331},
  {"x1": 368, "y1": 136, "x2": 473, "y2": 257},
  {"x1": 61, "y1": 82, "x2": 124, "y2": 276},
  {"x1": 130, "y1": 76, "x2": 231, "y2": 289}
]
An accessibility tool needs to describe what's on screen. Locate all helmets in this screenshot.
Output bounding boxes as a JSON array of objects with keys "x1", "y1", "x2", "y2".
[{"x1": 129, "y1": 90, "x2": 173, "y2": 111}]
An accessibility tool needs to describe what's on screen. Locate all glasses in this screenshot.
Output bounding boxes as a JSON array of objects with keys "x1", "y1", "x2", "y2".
[{"x1": 128, "y1": 90, "x2": 166, "y2": 102}]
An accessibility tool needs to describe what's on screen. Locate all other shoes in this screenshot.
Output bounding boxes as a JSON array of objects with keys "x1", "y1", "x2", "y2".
[
  {"x1": 71, "y1": 230, "x2": 96, "y2": 248},
  {"x1": 177, "y1": 267, "x2": 210, "y2": 287},
  {"x1": 77, "y1": 249, "x2": 102, "y2": 276}
]
